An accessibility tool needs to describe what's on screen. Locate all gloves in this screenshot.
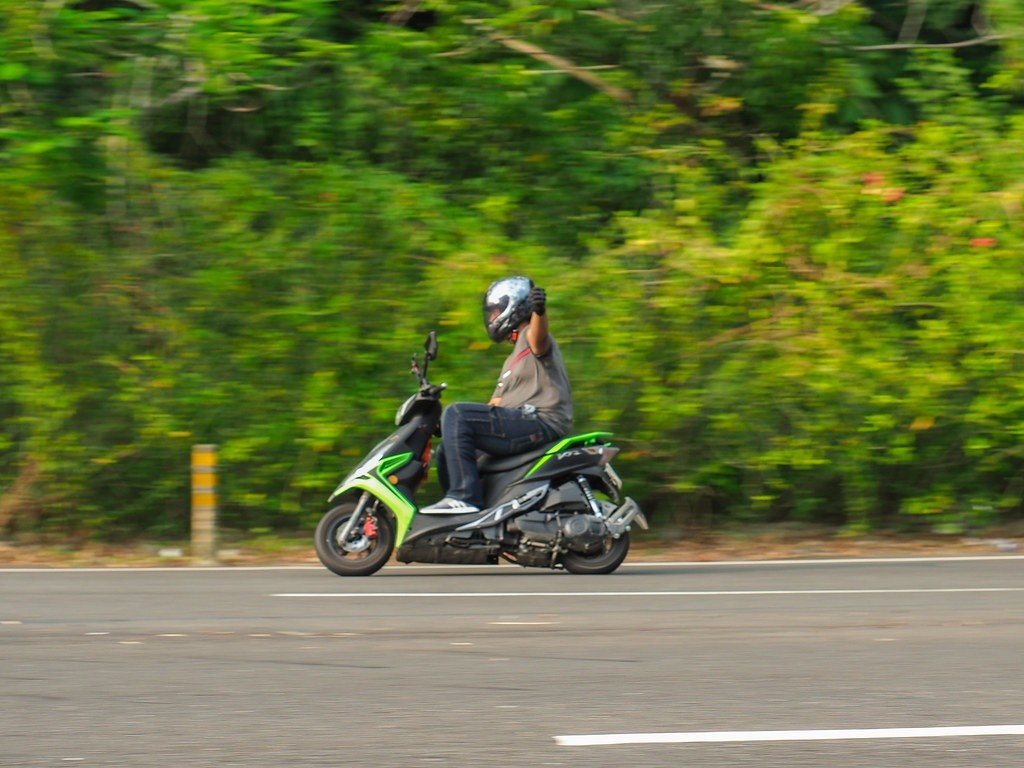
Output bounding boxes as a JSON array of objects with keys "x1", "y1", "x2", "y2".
[{"x1": 529, "y1": 280, "x2": 546, "y2": 316}]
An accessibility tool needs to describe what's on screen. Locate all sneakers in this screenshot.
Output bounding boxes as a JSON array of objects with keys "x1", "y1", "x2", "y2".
[{"x1": 420, "y1": 497, "x2": 480, "y2": 514}]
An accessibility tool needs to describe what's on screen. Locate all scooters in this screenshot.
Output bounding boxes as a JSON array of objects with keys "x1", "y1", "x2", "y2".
[{"x1": 312, "y1": 330, "x2": 650, "y2": 578}]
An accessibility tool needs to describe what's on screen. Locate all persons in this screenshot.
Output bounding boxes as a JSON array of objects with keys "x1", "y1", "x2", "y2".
[{"x1": 417, "y1": 277, "x2": 573, "y2": 516}]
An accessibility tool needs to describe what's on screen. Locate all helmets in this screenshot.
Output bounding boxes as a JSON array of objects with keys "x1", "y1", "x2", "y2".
[{"x1": 483, "y1": 276, "x2": 534, "y2": 342}]
